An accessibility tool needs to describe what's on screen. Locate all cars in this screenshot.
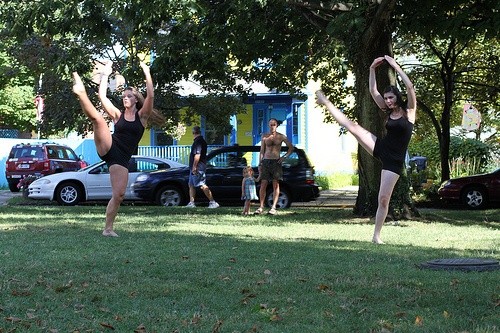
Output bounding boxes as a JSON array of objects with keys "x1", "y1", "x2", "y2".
[
  {"x1": 27, "y1": 155, "x2": 188, "y2": 206},
  {"x1": 438, "y1": 168, "x2": 500, "y2": 211}
]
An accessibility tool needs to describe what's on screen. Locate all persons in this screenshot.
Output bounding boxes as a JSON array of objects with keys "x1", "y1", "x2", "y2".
[
  {"x1": 72, "y1": 60, "x2": 168, "y2": 235},
  {"x1": 317, "y1": 55, "x2": 416, "y2": 245},
  {"x1": 254, "y1": 118, "x2": 294, "y2": 215},
  {"x1": 186, "y1": 126, "x2": 220, "y2": 208},
  {"x1": 241, "y1": 166, "x2": 260, "y2": 215}
]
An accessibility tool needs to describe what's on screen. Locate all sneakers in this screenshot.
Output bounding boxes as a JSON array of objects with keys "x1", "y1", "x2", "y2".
[
  {"x1": 208, "y1": 201, "x2": 220, "y2": 208},
  {"x1": 182, "y1": 203, "x2": 196, "y2": 208}
]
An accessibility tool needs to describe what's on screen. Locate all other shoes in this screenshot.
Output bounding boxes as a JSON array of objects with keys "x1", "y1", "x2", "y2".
[
  {"x1": 254, "y1": 208, "x2": 263, "y2": 214},
  {"x1": 269, "y1": 208, "x2": 277, "y2": 214}
]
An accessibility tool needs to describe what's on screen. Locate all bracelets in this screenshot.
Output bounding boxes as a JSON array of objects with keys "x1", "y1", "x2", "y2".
[{"x1": 284, "y1": 156, "x2": 286, "y2": 158}]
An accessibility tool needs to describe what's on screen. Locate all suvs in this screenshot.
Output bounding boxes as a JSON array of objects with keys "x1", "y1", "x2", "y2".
[
  {"x1": 5, "y1": 143, "x2": 90, "y2": 193},
  {"x1": 131, "y1": 145, "x2": 321, "y2": 210}
]
